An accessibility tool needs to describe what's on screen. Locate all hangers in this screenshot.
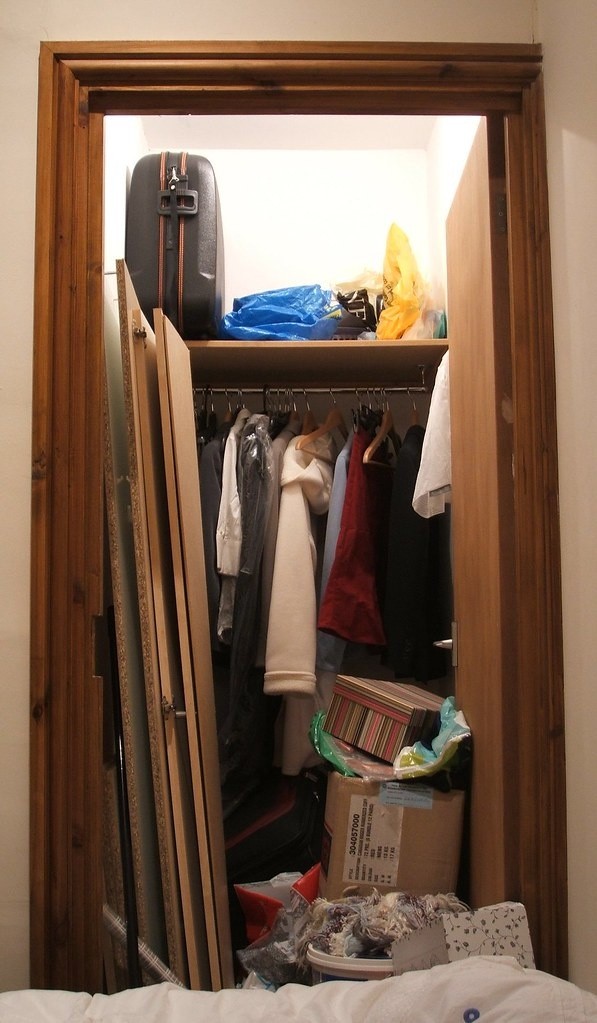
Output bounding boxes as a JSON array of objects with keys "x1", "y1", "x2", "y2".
[{"x1": 192, "y1": 385, "x2": 421, "y2": 468}]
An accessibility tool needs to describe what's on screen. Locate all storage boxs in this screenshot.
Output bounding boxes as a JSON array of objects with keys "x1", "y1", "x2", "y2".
[
  {"x1": 318, "y1": 770, "x2": 464, "y2": 902},
  {"x1": 390, "y1": 901, "x2": 536, "y2": 976},
  {"x1": 322, "y1": 674, "x2": 446, "y2": 764}
]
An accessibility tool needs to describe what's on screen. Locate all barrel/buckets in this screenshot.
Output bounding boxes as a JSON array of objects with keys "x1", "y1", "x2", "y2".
[{"x1": 306, "y1": 943, "x2": 396, "y2": 987}]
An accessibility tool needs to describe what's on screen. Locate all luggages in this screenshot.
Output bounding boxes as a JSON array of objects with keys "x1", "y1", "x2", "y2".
[{"x1": 125, "y1": 150, "x2": 223, "y2": 338}]
